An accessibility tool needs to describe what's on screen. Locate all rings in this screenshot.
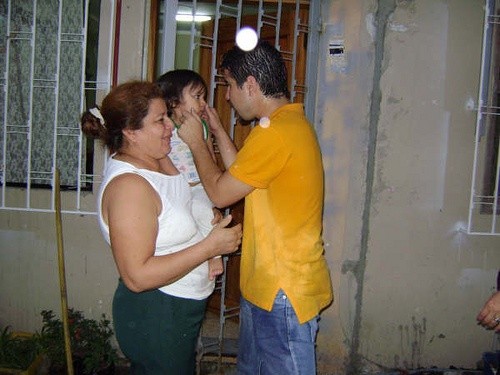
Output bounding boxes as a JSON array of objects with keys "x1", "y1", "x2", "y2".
[{"x1": 495, "y1": 317, "x2": 500, "y2": 323}]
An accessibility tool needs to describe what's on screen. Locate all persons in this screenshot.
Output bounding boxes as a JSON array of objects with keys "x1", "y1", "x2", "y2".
[
  {"x1": 152, "y1": 69, "x2": 228, "y2": 281},
  {"x1": 80, "y1": 80, "x2": 218, "y2": 375},
  {"x1": 176, "y1": 39, "x2": 334, "y2": 375},
  {"x1": 475, "y1": 271, "x2": 500, "y2": 375}
]
